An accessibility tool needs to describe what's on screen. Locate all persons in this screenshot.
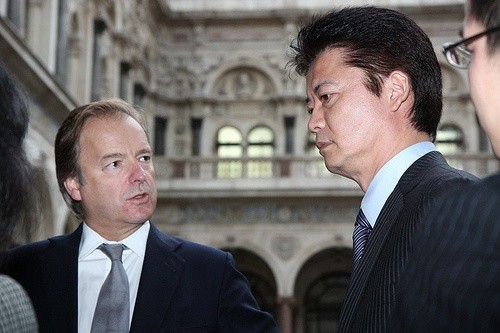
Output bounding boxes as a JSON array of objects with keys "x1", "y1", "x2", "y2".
[
  {"x1": 0, "y1": 98, "x2": 279, "y2": 333},
  {"x1": 388, "y1": 0, "x2": 500, "y2": 333},
  {"x1": 0, "y1": 70, "x2": 50, "y2": 333},
  {"x1": 288, "y1": 4, "x2": 482, "y2": 333}
]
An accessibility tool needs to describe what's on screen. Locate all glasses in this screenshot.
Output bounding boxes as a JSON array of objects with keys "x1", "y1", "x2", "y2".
[{"x1": 443, "y1": 25, "x2": 500, "y2": 70}]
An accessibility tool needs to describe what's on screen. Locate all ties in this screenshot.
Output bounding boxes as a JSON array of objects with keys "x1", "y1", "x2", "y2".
[
  {"x1": 352, "y1": 208, "x2": 372, "y2": 275},
  {"x1": 90, "y1": 244, "x2": 130, "y2": 333}
]
